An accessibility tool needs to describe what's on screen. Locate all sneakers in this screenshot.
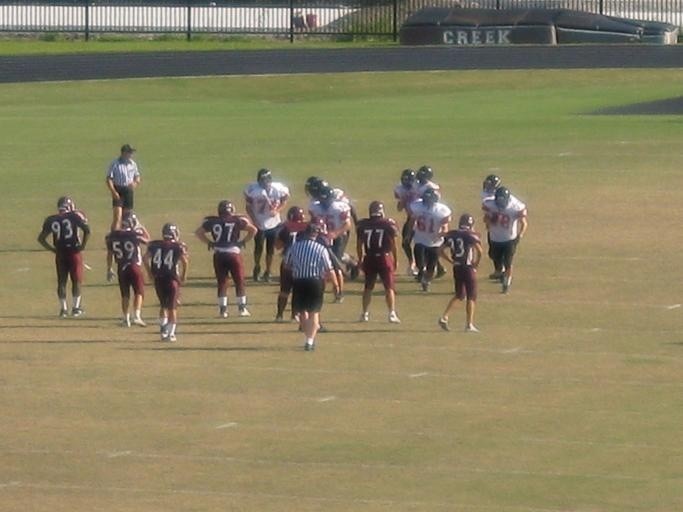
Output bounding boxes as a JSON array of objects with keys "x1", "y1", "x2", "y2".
[
  {"x1": 304, "y1": 342, "x2": 315, "y2": 351},
  {"x1": 254, "y1": 266, "x2": 261, "y2": 280},
  {"x1": 264, "y1": 272, "x2": 272, "y2": 283},
  {"x1": 72, "y1": 306, "x2": 85, "y2": 317},
  {"x1": 467, "y1": 323, "x2": 477, "y2": 332},
  {"x1": 389, "y1": 311, "x2": 401, "y2": 322},
  {"x1": 169, "y1": 333, "x2": 176, "y2": 342},
  {"x1": 276, "y1": 313, "x2": 283, "y2": 320},
  {"x1": 335, "y1": 292, "x2": 343, "y2": 303},
  {"x1": 489, "y1": 271, "x2": 511, "y2": 294},
  {"x1": 60, "y1": 309, "x2": 69, "y2": 319},
  {"x1": 161, "y1": 329, "x2": 170, "y2": 339},
  {"x1": 220, "y1": 307, "x2": 229, "y2": 318},
  {"x1": 408, "y1": 264, "x2": 448, "y2": 291},
  {"x1": 240, "y1": 305, "x2": 251, "y2": 316},
  {"x1": 361, "y1": 311, "x2": 369, "y2": 322},
  {"x1": 351, "y1": 263, "x2": 359, "y2": 278},
  {"x1": 133, "y1": 319, "x2": 146, "y2": 326},
  {"x1": 439, "y1": 318, "x2": 451, "y2": 331},
  {"x1": 123, "y1": 321, "x2": 132, "y2": 327}
]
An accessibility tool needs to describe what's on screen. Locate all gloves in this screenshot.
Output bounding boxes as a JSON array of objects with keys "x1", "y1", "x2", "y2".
[
  {"x1": 207, "y1": 240, "x2": 215, "y2": 251},
  {"x1": 237, "y1": 240, "x2": 247, "y2": 250},
  {"x1": 515, "y1": 234, "x2": 522, "y2": 246},
  {"x1": 107, "y1": 269, "x2": 118, "y2": 282}
]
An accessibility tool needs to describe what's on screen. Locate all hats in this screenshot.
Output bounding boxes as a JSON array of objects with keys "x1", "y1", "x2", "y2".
[{"x1": 121, "y1": 144, "x2": 136, "y2": 152}]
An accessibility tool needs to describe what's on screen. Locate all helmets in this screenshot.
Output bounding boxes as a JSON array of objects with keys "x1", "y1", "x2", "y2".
[
  {"x1": 417, "y1": 166, "x2": 433, "y2": 179},
  {"x1": 257, "y1": 168, "x2": 273, "y2": 180},
  {"x1": 218, "y1": 200, "x2": 234, "y2": 215},
  {"x1": 311, "y1": 216, "x2": 328, "y2": 229},
  {"x1": 369, "y1": 201, "x2": 384, "y2": 216},
  {"x1": 304, "y1": 176, "x2": 334, "y2": 205},
  {"x1": 495, "y1": 187, "x2": 510, "y2": 200},
  {"x1": 401, "y1": 169, "x2": 415, "y2": 185},
  {"x1": 57, "y1": 196, "x2": 74, "y2": 213},
  {"x1": 162, "y1": 223, "x2": 179, "y2": 240},
  {"x1": 483, "y1": 175, "x2": 502, "y2": 193},
  {"x1": 120, "y1": 212, "x2": 138, "y2": 229},
  {"x1": 423, "y1": 188, "x2": 439, "y2": 202},
  {"x1": 288, "y1": 207, "x2": 305, "y2": 221},
  {"x1": 459, "y1": 214, "x2": 474, "y2": 229}
]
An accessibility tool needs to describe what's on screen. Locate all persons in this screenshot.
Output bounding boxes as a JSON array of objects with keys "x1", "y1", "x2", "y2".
[
  {"x1": 104, "y1": 212, "x2": 148, "y2": 326},
  {"x1": 481, "y1": 175, "x2": 526, "y2": 293},
  {"x1": 394, "y1": 165, "x2": 451, "y2": 292},
  {"x1": 357, "y1": 200, "x2": 400, "y2": 323},
  {"x1": 244, "y1": 167, "x2": 290, "y2": 283},
  {"x1": 274, "y1": 176, "x2": 359, "y2": 348},
  {"x1": 143, "y1": 224, "x2": 188, "y2": 343},
  {"x1": 195, "y1": 200, "x2": 258, "y2": 318},
  {"x1": 439, "y1": 213, "x2": 482, "y2": 333},
  {"x1": 37, "y1": 195, "x2": 90, "y2": 317},
  {"x1": 105, "y1": 142, "x2": 140, "y2": 231}
]
